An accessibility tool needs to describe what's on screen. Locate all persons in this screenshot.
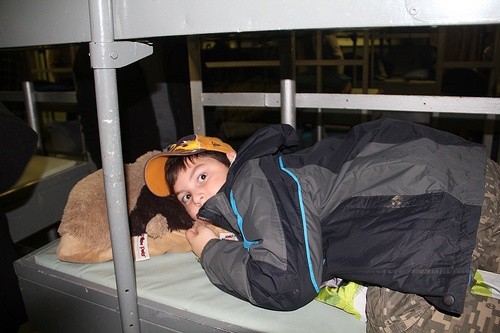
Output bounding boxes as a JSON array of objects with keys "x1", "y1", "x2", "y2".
[
  {"x1": 142, "y1": 117, "x2": 500, "y2": 333},
  {"x1": 71, "y1": 43, "x2": 162, "y2": 173}
]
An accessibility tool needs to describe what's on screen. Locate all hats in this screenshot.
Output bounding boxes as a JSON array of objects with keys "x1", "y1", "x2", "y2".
[{"x1": 144, "y1": 135, "x2": 235, "y2": 197}]
